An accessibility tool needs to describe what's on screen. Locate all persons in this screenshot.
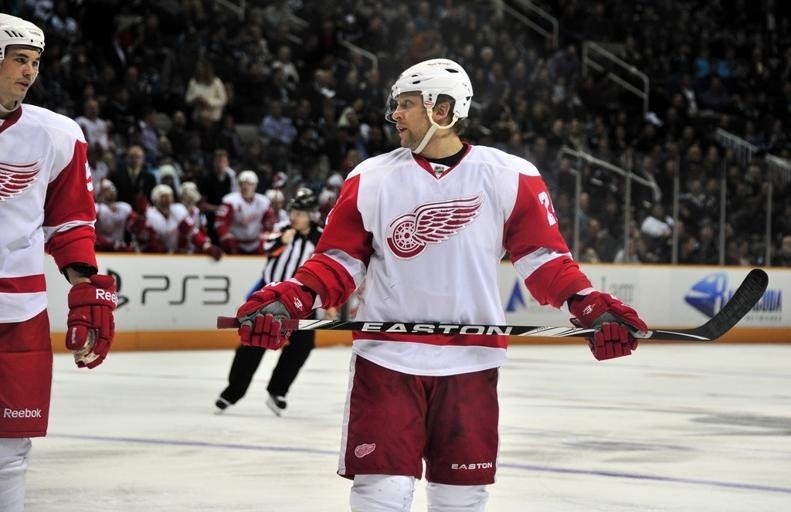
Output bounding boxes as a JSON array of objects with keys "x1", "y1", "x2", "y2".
[
  {"x1": 0, "y1": 13, "x2": 117, "y2": 511},
  {"x1": 235, "y1": 60, "x2": 645, "y2": 512},
  {"x1": 0, "y1": 0, "x2": 791, "y2": 270},
  {"x1": 213, "y1": 194, "x2": 325, "y2": 417}
]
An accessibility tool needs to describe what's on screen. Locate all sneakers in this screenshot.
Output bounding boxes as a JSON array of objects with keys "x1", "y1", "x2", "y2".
[
  {"x1": 216, "y1": 389, "x2": 234, "y2": 410},
  {"x1": 267, "y1": 387, "x2": 287, "y2": 410}
]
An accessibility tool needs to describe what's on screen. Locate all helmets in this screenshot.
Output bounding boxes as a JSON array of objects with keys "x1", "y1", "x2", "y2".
[
  {"x1": 265, "y1": 189, "x2": 285, "y2": 203},
  {"x1": 238, "y1": 169, "x2": 259, "y2": 184},
  {"x1": 384, "y1": 56, "x2": 475, "y2": 130},
  {"x1": 0, "y1": 12, "x2": 46, "y2": 62},
  {"x1": 94, "y1": 177, "x2": 117, "y2": 200},
  {"x1": 151, "y1": 164, "x2": 201, "y2": 204},
  {"x1": 289, "y1": 173, "x2": 348, "y2": 211}
]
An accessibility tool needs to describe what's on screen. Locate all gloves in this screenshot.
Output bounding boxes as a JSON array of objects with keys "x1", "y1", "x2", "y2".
[
  {"x1": 202, "y1": 241, "x2": 222, "y2": 260},
  {"x1": 235, "y1": 280, "x2": 316, "y2": 353},
  {"x1": 64, "y1": 272, "x2": 119, "y2": 370},
  {"x1": 569, "y1": 291, "x2": 648, "y2": 362}
]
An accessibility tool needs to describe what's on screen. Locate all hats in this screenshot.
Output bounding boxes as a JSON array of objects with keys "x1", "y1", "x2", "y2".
[{"x1": 644, "y1": 111, "x2": 661, "y2": 126}]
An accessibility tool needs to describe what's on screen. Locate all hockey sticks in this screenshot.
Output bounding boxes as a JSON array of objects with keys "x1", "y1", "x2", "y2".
[{"x1": 218, "y1": 268, "x2": 769, "y2": 342}]
